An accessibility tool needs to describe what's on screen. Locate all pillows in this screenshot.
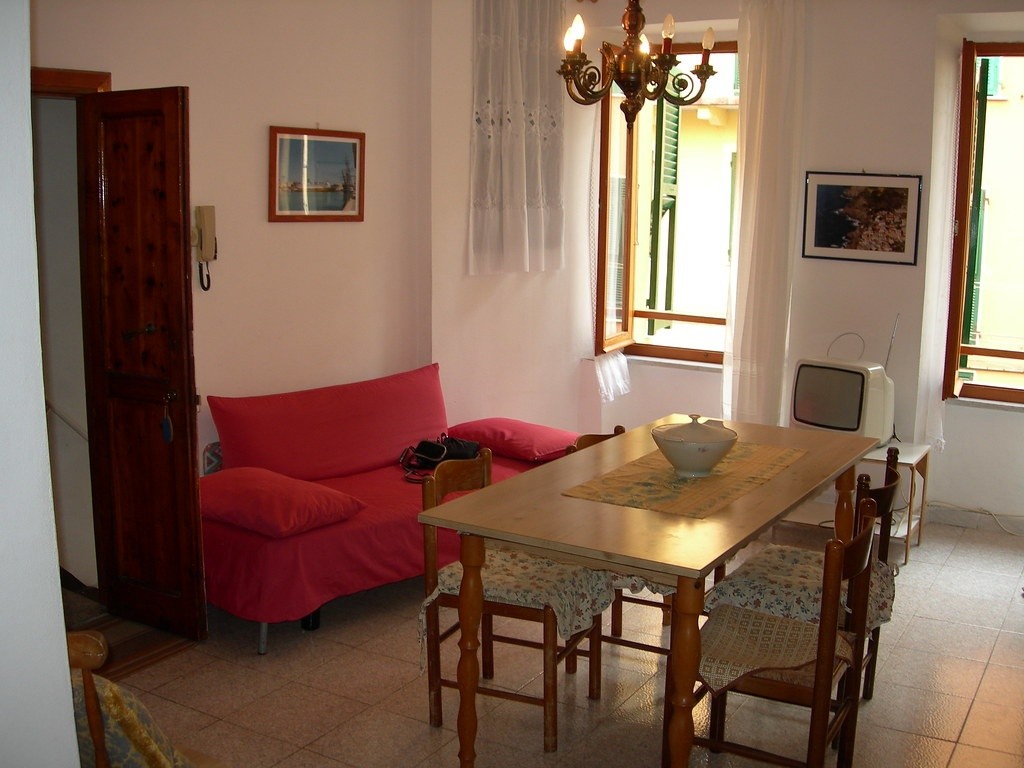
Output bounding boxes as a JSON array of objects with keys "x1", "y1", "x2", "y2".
[
  {"x1": 200, "y1": 467, "x2": 370, "y2": 538},
  {"x1": 446, "y1": 417, "x2": 581, "y2": 462},
  {"x1": 71, "y1": 669, "x2": 199, "y2": 768}
]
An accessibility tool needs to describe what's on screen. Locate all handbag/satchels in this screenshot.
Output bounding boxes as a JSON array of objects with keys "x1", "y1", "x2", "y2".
[{"x1": 400, "y1": 432, "x2": 480, "y2": 484}]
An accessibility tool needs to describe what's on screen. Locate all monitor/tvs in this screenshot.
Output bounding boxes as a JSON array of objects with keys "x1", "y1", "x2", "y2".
[{"x1": 789, "y1": 314, "x2": 899, "y2": 447}]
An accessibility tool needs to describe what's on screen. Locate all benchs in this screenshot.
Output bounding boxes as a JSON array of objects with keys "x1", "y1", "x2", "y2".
[{"x1": 199, "y1": 363, "x2": 582, "y2": 655}]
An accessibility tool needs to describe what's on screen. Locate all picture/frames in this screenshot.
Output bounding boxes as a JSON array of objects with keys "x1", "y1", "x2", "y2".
[
  {"x1": 268, "y1": 125, "x2": 365, "y2": 223},
  {"x1": 802, "y1": 171, "x2": 924, "y2": 267}
]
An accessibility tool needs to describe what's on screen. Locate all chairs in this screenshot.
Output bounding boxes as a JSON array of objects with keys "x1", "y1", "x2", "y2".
[
  {"x1": 421, "y1": 448, "x2": 602, "y2": 753},
  {"x1": 703, "y1": 447, "x2": 901, "y2": 750},
  {"x1": 66, "y1": 629, "x2": 113, "y2": 768},
  {"x1": 565, "y1": 425, "x2": 726, "y2": 674},
  {"x1": 690, "y1": 495, "x2": 876, "y2": 768}
]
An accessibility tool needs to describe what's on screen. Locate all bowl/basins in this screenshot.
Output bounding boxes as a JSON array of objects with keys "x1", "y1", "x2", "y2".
[{"x1": 651, "y1": 414, "x2": 739, "y2": 477}]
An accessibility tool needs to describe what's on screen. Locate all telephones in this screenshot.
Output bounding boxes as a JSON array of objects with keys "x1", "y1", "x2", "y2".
[{"x1": 195, "y1": 205, "x2": 216, "y2": 262}]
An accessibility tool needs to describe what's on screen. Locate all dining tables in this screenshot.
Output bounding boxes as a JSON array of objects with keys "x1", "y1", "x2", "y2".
[{"x1": 418, "y1": 413, "x2": 882, "y2": 767}]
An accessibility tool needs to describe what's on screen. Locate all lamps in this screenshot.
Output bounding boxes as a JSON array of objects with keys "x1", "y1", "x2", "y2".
[{"x1": 555, "y1": 0, "x2": 717, "y2": 136}]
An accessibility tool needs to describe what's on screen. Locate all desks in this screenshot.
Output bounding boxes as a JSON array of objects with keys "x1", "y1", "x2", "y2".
[{"x1": 771, "y1": 442, "x2": 932, "y2": 565}]
